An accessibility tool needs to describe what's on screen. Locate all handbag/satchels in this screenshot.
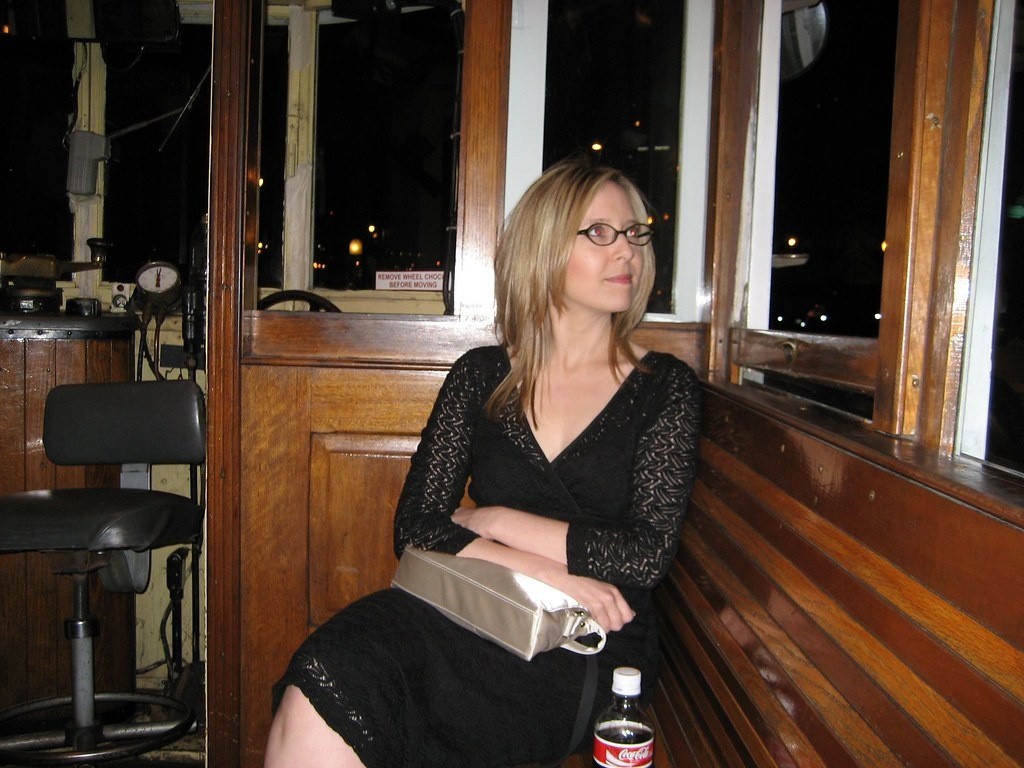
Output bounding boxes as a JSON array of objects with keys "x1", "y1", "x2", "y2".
[{"x1": 392, "y1": 546, "x2": 606, "y2": 661}]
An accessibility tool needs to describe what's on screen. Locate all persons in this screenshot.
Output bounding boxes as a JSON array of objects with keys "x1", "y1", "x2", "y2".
[{"x1": 262, "y1": 163, "x2": 703, "y2": 768}]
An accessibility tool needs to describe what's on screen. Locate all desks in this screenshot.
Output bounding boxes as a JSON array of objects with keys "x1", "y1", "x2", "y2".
[{"x1": 0, "y1": 313, "x2": 143, "y2": 740}]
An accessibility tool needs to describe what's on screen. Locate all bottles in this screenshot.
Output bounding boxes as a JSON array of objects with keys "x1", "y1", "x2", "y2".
[{"x1": 592, "y1": 667, "x2": 654, "y2": 768}]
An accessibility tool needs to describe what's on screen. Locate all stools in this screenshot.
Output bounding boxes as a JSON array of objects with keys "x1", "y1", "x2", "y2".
[{"x1": 0, "y1": 378, "x2": 206, "y2": 768}]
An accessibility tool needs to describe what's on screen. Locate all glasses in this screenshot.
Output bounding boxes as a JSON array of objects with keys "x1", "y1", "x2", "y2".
[{"x1": 577, "y1": 223, "x2": 657, "y2": 246}]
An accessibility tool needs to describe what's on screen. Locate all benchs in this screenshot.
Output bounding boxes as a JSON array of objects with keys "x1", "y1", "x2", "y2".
[{"x1": 558, "y1": 383, "x2": 1024, "y2": 768}]
